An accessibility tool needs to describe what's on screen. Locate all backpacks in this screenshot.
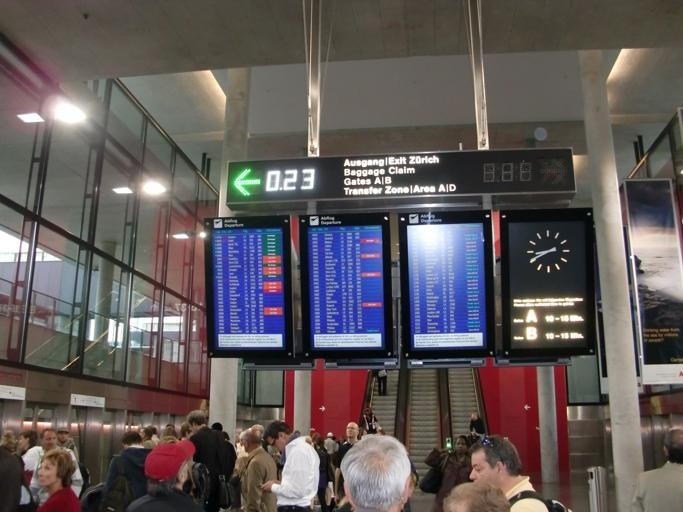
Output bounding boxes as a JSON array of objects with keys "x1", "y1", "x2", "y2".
[
  {"x1": 507, "y1": 491, "x2": 572, "y2": 512},
  {"x1": 102, "y1": 455, "x2": 136, "y2": 512}
]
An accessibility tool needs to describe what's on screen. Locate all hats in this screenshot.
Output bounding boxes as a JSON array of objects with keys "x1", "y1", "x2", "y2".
[
  {"x1": 144, "y1": 440, "x2": 195, "y2": 481},
  {"x1": 58, "y1": 427, "x2": 70, "y2": 433}
]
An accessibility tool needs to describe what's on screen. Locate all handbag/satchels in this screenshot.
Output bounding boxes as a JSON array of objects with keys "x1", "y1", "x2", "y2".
[
  {"x1": 419, "y1": 468, "x2": 443, "y2": 493},
  {"x1": 191, "y1": 462, "x2": 242, "y2": 509}
]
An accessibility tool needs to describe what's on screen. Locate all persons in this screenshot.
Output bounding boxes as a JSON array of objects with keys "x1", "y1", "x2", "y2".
[
  {"x1": 2, "y1": 408, "x2": 549, "y2": 511},
  {"x1": 631, "y1": 429, "x2": 683, "y2": 511},
  {"x1": 377, "y1": 368, "x2": 387, "y2": 395}
]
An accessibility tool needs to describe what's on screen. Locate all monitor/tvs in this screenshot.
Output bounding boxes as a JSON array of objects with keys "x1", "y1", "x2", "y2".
[
  {"x1": 397, "y1": 209, "x2": 495, "y2": 359},
  {"x1": 499, "y1": 207, "x2": 596, "y2": 359},
  {"x1": 202, "y1": 216, "x2": 294, "y2": 359},
  {"x1": 298, "y1": 212, "x2": 395, "y2": 360}
]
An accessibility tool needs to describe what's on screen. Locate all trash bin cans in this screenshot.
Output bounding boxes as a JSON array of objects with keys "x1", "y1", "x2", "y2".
[{"x1": 585, "y1": 465, "x2": 609, "y2": 512}]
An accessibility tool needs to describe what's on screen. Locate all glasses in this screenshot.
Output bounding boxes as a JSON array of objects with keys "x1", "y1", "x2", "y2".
[{"x1": 481, "y1": 433, "x2": 493, "y2": 448}]
